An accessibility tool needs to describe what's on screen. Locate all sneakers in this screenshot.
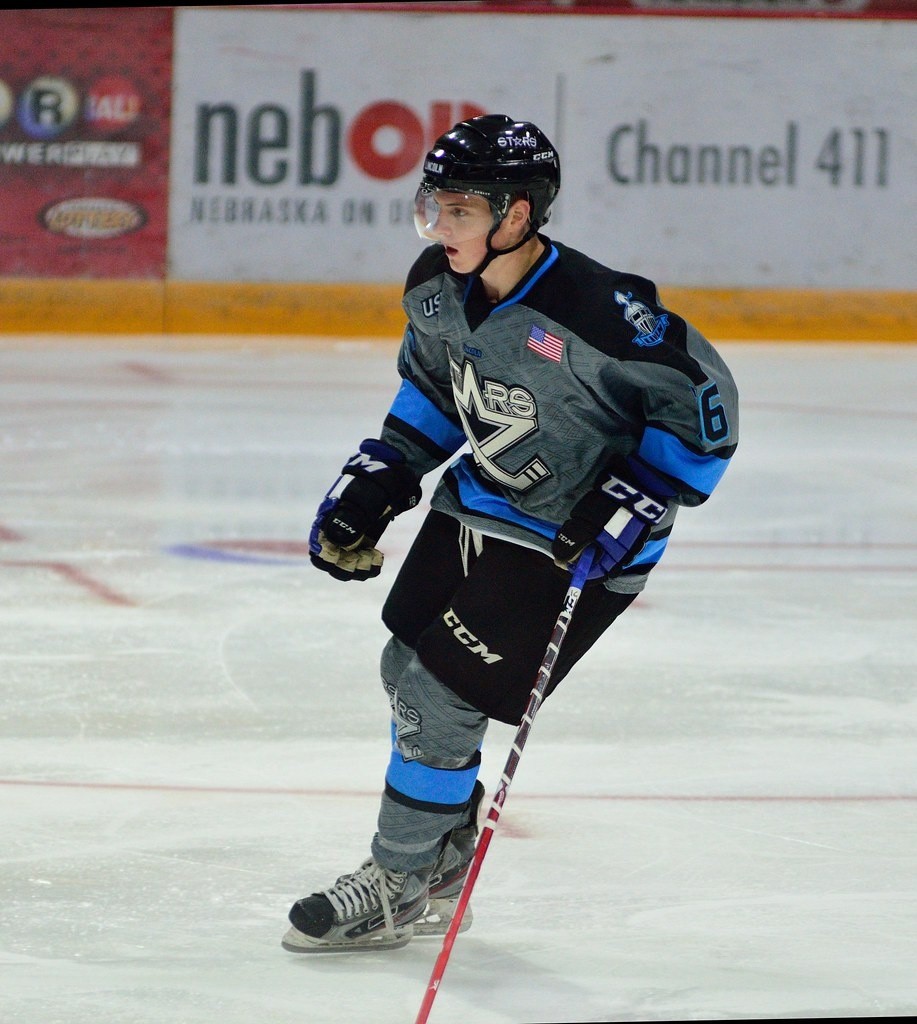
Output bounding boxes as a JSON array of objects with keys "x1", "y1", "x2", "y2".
[
  {"x1": 385, "y1": 779, "x2": 486, "y2": 936},
  {"x1": 281, "y1": 855, "x2": 429, "y2": 955}
]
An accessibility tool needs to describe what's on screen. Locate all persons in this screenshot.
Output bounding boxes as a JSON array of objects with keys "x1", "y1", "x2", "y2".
[{"x1": 279, "y1": 115, "x2": 738, "y2": 952}]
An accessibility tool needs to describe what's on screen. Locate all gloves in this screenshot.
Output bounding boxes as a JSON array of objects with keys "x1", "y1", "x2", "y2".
[
  {"x1": 552, "y1": 449, "x2": 676, "y2": 589},
  {"x1": 307, "y1": 437, "x2": 422, "y2": 581}
]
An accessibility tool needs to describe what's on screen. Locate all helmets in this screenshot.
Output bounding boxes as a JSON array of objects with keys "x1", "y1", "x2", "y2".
[{"x1": 423, "y1": 114, "x2": 560, "y2": 224}]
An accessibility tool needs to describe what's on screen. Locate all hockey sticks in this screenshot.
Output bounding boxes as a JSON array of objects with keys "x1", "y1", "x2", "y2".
[{"x1": 412, "y1": 544, "x2": 599, "y2": 1024}]
